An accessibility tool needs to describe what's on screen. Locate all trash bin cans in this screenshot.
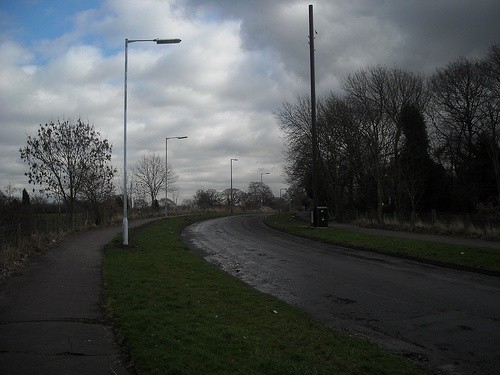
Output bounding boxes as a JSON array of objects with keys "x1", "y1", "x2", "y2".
[{"x1": 310, "y1": 206, "x2": 329, "y2": 227}]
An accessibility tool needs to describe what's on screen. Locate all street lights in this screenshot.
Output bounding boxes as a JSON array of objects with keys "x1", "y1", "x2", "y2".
[
  {"x1": 260, "y1": 172, "x2": 269, "y2": 212},
  {"x1": 164, "y1": 136, "x2": 186, "y2": 219},
  {"x1": 231, "y1": 158, "x2": 240, "y2": 215},
  {"x1": 120, "y1": 37, "x2": 183, "y2": 247}
]
